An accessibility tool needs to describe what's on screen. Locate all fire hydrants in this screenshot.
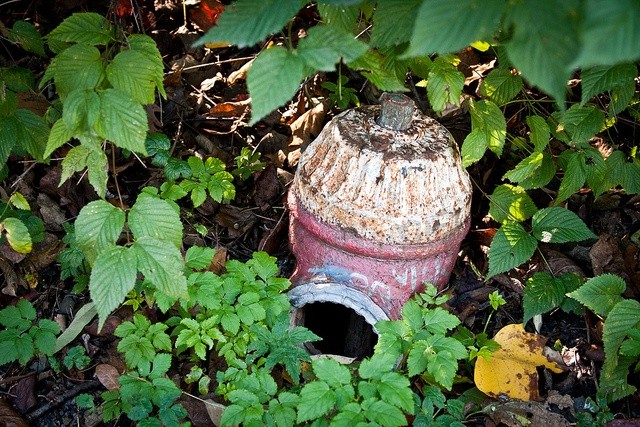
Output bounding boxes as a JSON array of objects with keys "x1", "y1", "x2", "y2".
[{"x1": 278, "y1": 92, "x2": 475, "y2": 382}]
